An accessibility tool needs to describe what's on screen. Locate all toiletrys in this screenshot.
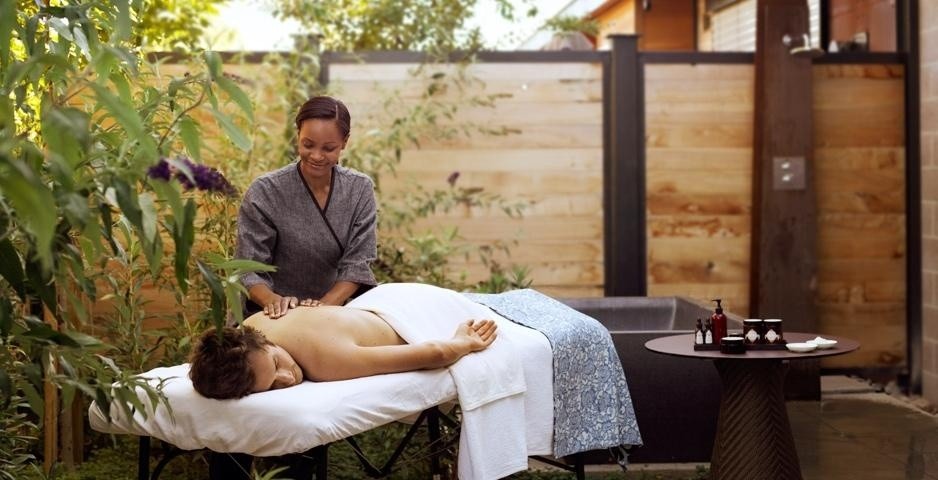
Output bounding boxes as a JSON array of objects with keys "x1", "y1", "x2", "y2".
[{"x1": 693, "y1": 297, "x2": 728, "y2": 350}]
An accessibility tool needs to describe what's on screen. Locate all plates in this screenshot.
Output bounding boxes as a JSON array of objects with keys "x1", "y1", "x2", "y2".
[
  {"x1": 807, "y1": 340, "x2": 837, "y2": 349},
  {"x1": 786, "y1": 343, "x2": 818, "y2": 353}
]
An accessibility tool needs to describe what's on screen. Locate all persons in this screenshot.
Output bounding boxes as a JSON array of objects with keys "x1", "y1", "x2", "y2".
[
  {"x1": 235, "y1": 96, "x2": 377, "y2": 319},
  {"x1": 187, "y1": 304, "x2": 498, "y2": 400}
]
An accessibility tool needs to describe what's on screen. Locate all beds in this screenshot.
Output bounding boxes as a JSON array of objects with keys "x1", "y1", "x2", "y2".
[{"x1": 90, "y1": 293, "x2": 645, "y2": 480}]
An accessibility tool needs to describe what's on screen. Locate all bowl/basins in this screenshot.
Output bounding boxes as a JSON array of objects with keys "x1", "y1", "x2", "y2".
[{"x1": 720, "y1": 336, "x2": 746, "y2": 354}]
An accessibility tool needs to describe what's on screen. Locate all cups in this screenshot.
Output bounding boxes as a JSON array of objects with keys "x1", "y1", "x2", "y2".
[{"x1": 743, "y1": 319, "x2": 783, "y2": 345}]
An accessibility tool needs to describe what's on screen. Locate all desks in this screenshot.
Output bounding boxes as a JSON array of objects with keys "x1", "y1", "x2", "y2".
[{"x1": 643, "y1": 331, "x2": 860, "y2": 479}]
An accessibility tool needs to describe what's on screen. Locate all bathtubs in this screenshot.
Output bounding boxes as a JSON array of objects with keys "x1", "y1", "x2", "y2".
[{"x1": 547, "y1": 295, "x2": 753, "y2": 460}]
